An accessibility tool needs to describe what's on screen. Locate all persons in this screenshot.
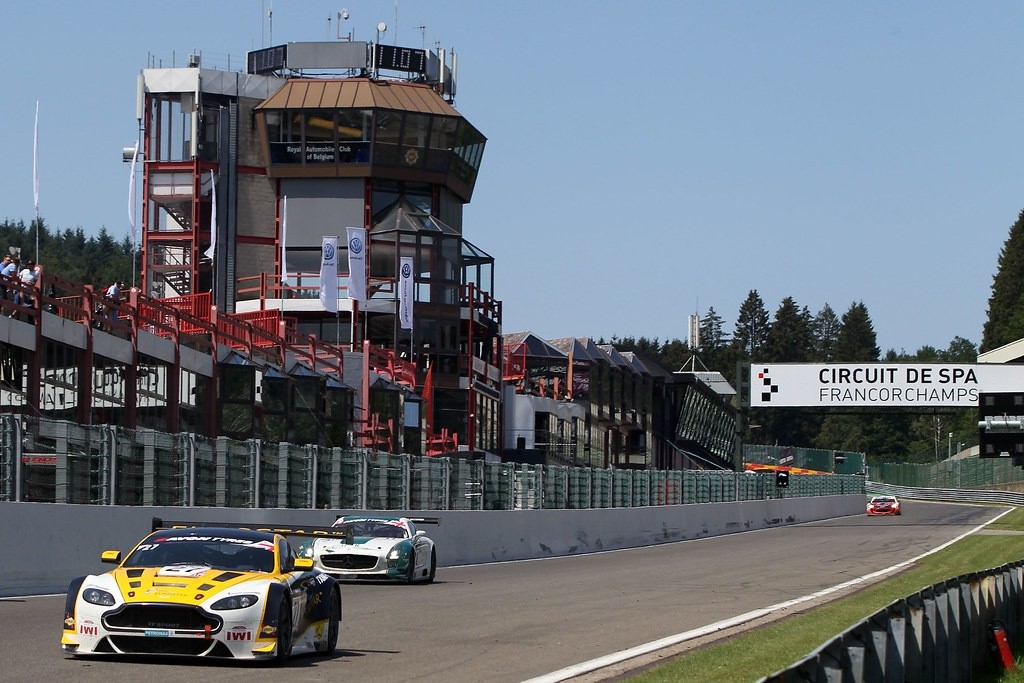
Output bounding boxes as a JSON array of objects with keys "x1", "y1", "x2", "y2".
[
  {"x1": 0, "y1": 255, "x2": 39, "y2": 318},
  {"x1": 93, "y1": 280, "x2": 122, "y2": 333}
]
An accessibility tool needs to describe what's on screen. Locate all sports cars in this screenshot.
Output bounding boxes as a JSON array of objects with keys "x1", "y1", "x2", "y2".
[
  {"x1": 305, "y1": 515, "x2": 443, "y2": 586},
  {"x1": 59, "y1": 515, "x2": 354, "y2": 667},
  {"x1": 867, "y1": 496, "x2": 901, "y2": 516}
]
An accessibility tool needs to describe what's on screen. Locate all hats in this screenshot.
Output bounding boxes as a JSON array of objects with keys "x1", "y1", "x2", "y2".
[{"x1": 26, "y1": 260, "x2": 35, "y2": 265}]
